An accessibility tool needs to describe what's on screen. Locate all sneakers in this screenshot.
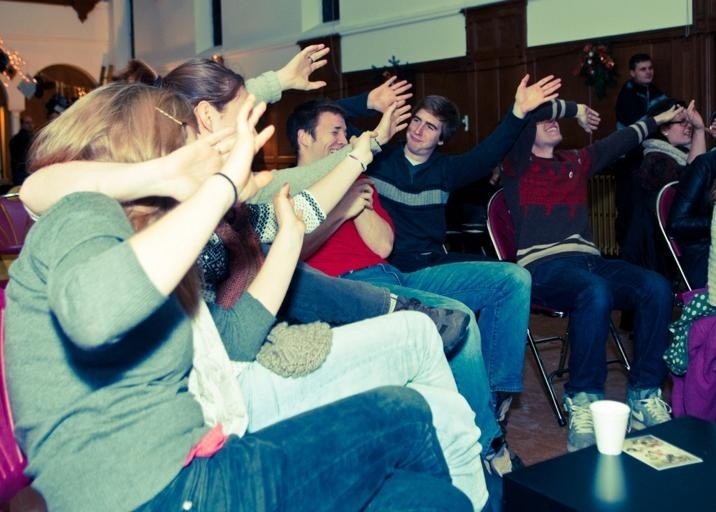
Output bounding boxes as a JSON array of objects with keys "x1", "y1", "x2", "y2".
[
  {"x1": 625, "y1": 397, "x2": 672, "y2": 433},
  {"x1": 485, "y1": 447, "x2": 524, "y2": 479},
  {"x1": 565, "y1": 392, "x2": 604, "y2": 453}
]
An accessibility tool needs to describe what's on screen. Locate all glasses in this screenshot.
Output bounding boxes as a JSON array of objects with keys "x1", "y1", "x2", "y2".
[
  {"x1": 671, "y1": 118, "x2": 691, "y2": 127},
  {"x1": 153, "y1": 104, "x2": 198, "y2": 143}
]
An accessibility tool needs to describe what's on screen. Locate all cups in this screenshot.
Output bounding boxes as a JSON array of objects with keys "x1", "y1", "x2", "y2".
[{"x1": 588, "y1": 399, "x2": 631, "y2": 456}]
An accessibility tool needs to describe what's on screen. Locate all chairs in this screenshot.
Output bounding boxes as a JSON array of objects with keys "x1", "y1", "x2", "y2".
[
  {"x1": 655, "y1": 180, "x2": 692, "y2": 293},
  {"x1": 486, "y1": 189, "x2": 632, "y2": 426}
]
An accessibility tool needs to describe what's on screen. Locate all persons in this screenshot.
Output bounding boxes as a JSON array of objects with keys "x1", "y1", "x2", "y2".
[
  {"x1": 1, "y1": 42, "x2": 561, "y2": 512},
  {"x1": 501, "y1": 53, "x2": 716, "y2": 453}
]
